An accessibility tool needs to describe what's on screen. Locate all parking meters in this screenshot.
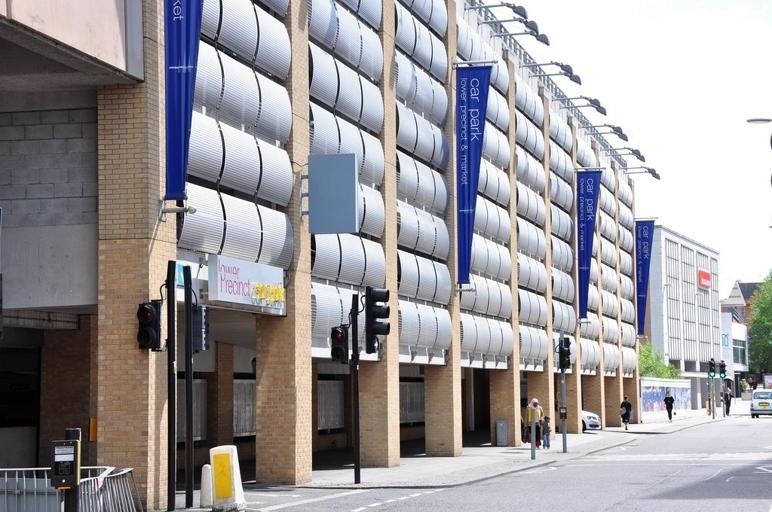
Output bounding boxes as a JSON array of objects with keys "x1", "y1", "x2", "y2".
[{"x1": 51, "y1": 426, "x2": 82, "y2": 512}]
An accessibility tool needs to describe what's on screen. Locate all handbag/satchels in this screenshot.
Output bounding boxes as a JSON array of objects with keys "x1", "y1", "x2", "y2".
[
  {"x1": 618, "y1": 407, "x2": 626, "y2": 415},
  {"x1": 522, "y1": 427, "x2": 528, "y2": 443}
]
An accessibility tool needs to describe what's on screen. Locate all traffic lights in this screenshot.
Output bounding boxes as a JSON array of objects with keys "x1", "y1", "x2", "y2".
[
  {"x1": 719, "y1": 360, "x2": 725, "y2": 379},
  {"x1": 560, "y1": 336, "x2": 571, "y2": 371},
  {"x1": 364, "y1": 286, "x2": 390, "y2": 354},
  {"x1": 709, "y1": 359, "x2": 715, "y2": 378},
  {"x1": 329, "y1": 326, "x2": 348, "y2": 362},
  {"x1": 137, "y1": 301, "x2": 161, "y2": 349}
]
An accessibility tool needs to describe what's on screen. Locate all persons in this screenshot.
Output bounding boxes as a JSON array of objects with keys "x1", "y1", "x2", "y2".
[
  {"x1": 724, "y1": 387, "x2": 734, "y2": 416},
  {"x1": 523, "y1": 397, "x2": 544, "y2": 448},
  {"x1": 742, "y1": 375, "x2": 762, "y2": 389},
  {"x1": 542, "y1": 415, "x2": 552, "y2": 449},
  {"x1": 619, "y1": 395, "x2": 632, "y2": 430},
  {"x1": 662, "y1": 390, "x2": 675, "y2": 422}
]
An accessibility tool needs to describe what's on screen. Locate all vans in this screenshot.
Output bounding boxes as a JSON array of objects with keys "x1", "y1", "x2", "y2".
[{"x1": 750, "y1": 389, "x2": 772, "y2": 418}]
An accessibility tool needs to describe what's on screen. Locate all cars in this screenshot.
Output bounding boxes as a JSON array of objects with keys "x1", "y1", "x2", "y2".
[{"x1": 521, "y1": 398, "x2": 602, "y2": 433}]
